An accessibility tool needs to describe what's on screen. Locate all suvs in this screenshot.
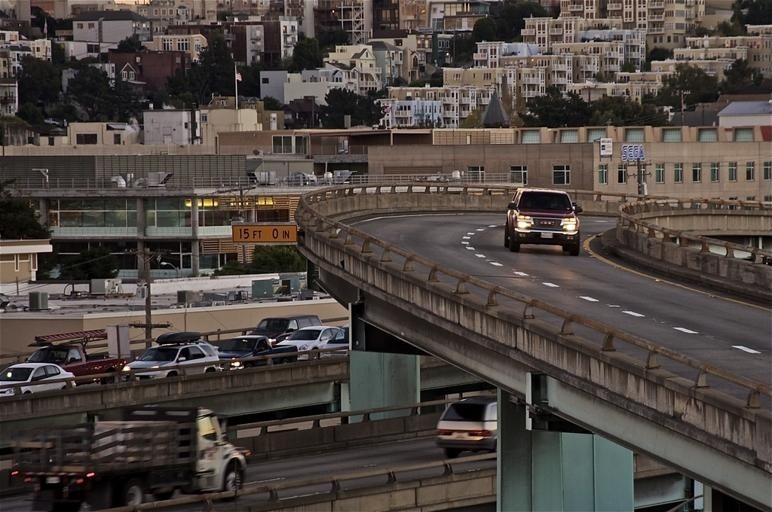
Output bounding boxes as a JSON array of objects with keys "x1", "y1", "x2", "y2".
[
  {"x1": 503, "y1": 185, "x2": 583, "y2": 257},
  {"x1": 120, "y1": 331, "x2": 224, "y2": 384},
  {"x1": 250, "y1": 314, "x2": 321, "y2": 347},
  {"x1": 435, "y1": 396, "x2": 497, "y2": 457}
]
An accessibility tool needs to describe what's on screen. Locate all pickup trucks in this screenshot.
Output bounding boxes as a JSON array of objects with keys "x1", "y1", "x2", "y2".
[
  {"x1": 27, "y1": 343, "x2": 131, "y2": 386},
  {"x1": 218, "y1": 335, "x2": 297, "y2": 371}
]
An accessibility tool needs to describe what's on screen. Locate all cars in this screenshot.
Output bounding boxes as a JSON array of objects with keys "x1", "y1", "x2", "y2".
[
  {"x1": 278, "y1": 326, "x2": 348, "y2": 359},
  {"x1": 0, "y1": 363, "x2": 77, "y2": 400}
]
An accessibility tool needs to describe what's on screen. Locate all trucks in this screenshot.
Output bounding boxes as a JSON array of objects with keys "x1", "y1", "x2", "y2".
[{"x1": 13, "y1": 399, "x2": 253, "y2": 509}]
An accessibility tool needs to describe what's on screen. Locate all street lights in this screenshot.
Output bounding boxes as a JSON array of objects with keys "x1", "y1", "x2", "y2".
[{"x1": 159, "y1": 261, "x2": 178, "y2": 279}]
